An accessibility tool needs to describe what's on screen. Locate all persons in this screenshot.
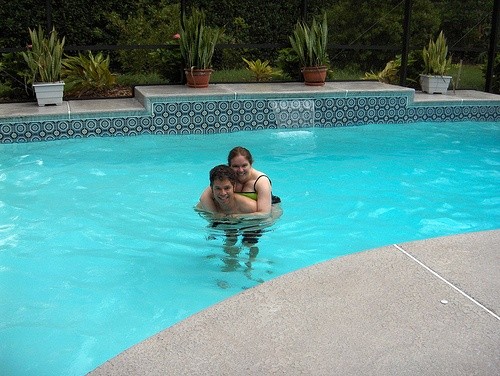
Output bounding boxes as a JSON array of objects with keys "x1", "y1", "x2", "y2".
[
  {"x1": 198, "y1": 165, "x2": 257, "y2": 213},
  {"x1": 200, "y1": 146, "x2": 272, "y2": 217}
]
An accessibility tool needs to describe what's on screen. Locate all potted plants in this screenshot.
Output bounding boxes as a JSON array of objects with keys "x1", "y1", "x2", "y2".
[
  {"x1": 288, "y1": 11, "x2": 330, "y2": 86},
  {"x1": 21, "y1": 26, "x2": 66, "y2": 107},
  {"x1": 419, "y1": 30, "x2": 453, "y2": 95},
  {"x1": 178, "y1": 7, "x2": 226, "y2": 89}
]
